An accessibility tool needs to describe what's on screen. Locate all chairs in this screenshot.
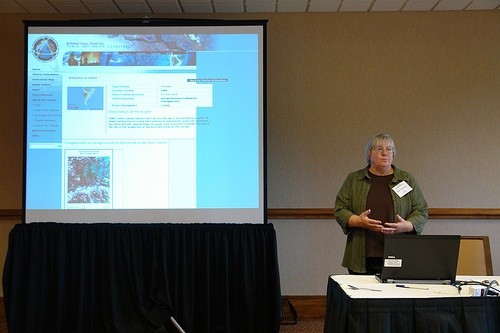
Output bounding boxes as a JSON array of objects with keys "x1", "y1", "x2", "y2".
[{"x1": 456, "y1": 236, "x2": 493, "y2": 277}]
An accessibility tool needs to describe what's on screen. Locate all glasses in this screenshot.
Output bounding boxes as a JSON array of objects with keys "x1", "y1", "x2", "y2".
[{"x1": 374, "y1": 146, "x2": 392, "y2": 153}]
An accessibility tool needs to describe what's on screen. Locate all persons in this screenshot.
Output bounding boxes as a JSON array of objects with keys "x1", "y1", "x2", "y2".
[
  {"x1": 84, "y1": 55, "x2": 88, "y2": 64},
  {"x1": 68, "y1": 53, "x2": 78, "y2": 66},
  {"x1": 334, "y1": 133, "x2": 428, "y2": 275}
]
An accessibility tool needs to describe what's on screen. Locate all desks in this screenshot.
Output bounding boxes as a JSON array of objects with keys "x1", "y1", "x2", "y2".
[{"x1": 324, "y1": 275, "x2": 500, "y2": 333}]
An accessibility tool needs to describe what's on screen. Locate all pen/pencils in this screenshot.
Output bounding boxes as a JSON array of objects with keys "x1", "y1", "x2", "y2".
[{"x1": 396, "y1": 284, "x2": 428, "y2": 291}]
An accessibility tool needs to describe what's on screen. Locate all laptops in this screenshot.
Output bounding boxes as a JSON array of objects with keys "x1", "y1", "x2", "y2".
[{"x1": 375, "y1": 233, "x2": 461, "y2": 283}]
